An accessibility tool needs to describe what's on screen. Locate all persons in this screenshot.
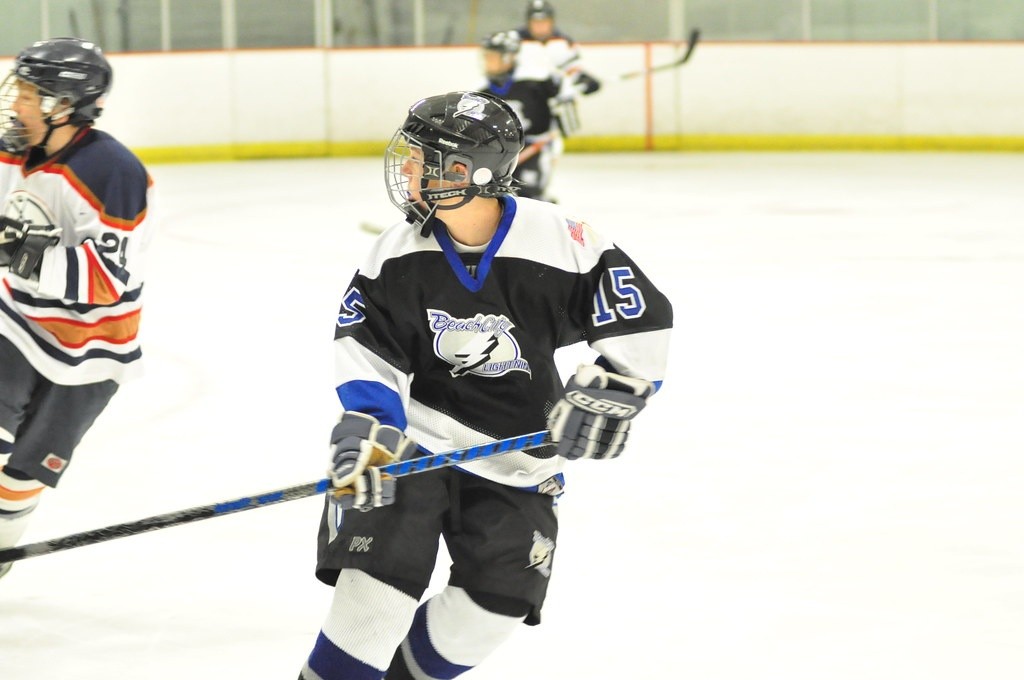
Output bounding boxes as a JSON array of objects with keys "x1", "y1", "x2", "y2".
[
  {"x1": 298, "y1": 92, "x2": 674, "y2": 680},
  {"x1": 1, "y1": 37, "x2": 154, "y2": 573},
  {"x1": 476, "y1": 0, "x2": 599, "y2": 201}
]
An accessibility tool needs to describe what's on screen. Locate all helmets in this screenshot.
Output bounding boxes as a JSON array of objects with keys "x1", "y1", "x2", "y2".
[
  {"x1": 0, "y1": 38, "x2": 114, "y2": 154},
  {"x1": 524, "y1": 0, "x2": 556, "y2": 41},
  {"x1": 483, "y1": 93, "x2": 485, "y2": 100},
  {"x1": 479, "y1": 27, "x2": 521, "y2": 81},
  {"x1": 384, "y1": 91, "x2": 527, "y2": 241}
]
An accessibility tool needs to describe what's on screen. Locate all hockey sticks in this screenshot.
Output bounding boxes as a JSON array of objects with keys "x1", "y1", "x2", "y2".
[
  {"x1": 579, "y1": 26, "x2": 702, "y2": 104},
  {"x1": 0, "y1": 427, "x2": 552, "y2": 577}
]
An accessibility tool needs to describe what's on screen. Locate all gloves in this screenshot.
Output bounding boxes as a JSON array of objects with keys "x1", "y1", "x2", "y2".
[
  {"x1": 0, "y1": 216, "x2": 65, "y2": 281},
  {"x1": 548, "y1": 362, "x2": 656, "y2": 461},
  {"x1": 325, "y1": 410, "x2": 418, "y2": 513}
]
